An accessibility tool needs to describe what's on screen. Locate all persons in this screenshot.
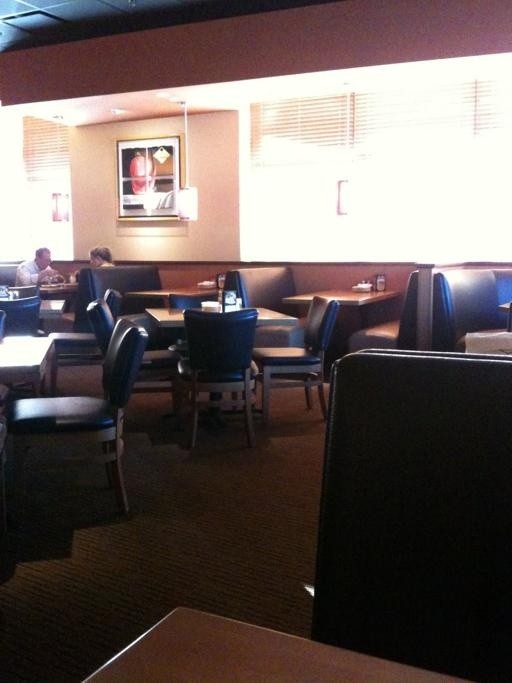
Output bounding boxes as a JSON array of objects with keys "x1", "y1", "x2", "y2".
[
  {"x1": 88, "y1": 246, "x2": 115, "y2": 267},
  {"x1": 14, "y1": 247, "x2": 58, "y2": 287}
]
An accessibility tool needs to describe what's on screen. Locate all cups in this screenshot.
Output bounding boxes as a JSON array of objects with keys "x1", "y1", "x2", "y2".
[{"x1": 201, "y1": 301, "x2": 222, "y2": 313}]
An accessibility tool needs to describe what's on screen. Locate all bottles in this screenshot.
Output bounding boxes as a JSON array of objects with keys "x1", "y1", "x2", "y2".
[{"x1": 376, "y1": 272, "x2": 385, "y2": 290}]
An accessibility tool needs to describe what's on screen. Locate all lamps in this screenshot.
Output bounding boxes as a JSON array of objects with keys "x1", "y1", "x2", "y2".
[{"x1": 176, "y1": 100, "x2": 199, "y2": 221}]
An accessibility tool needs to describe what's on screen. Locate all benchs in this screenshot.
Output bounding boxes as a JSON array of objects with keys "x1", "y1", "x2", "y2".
[{"x1": 309, "y1": 348, "x2": 511, "y2": 682}]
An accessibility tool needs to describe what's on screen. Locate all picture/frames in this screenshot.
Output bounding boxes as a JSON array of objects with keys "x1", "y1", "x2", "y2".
[{"x1": 111, "y1": 132, "x2": 186, "y2": 221}]
